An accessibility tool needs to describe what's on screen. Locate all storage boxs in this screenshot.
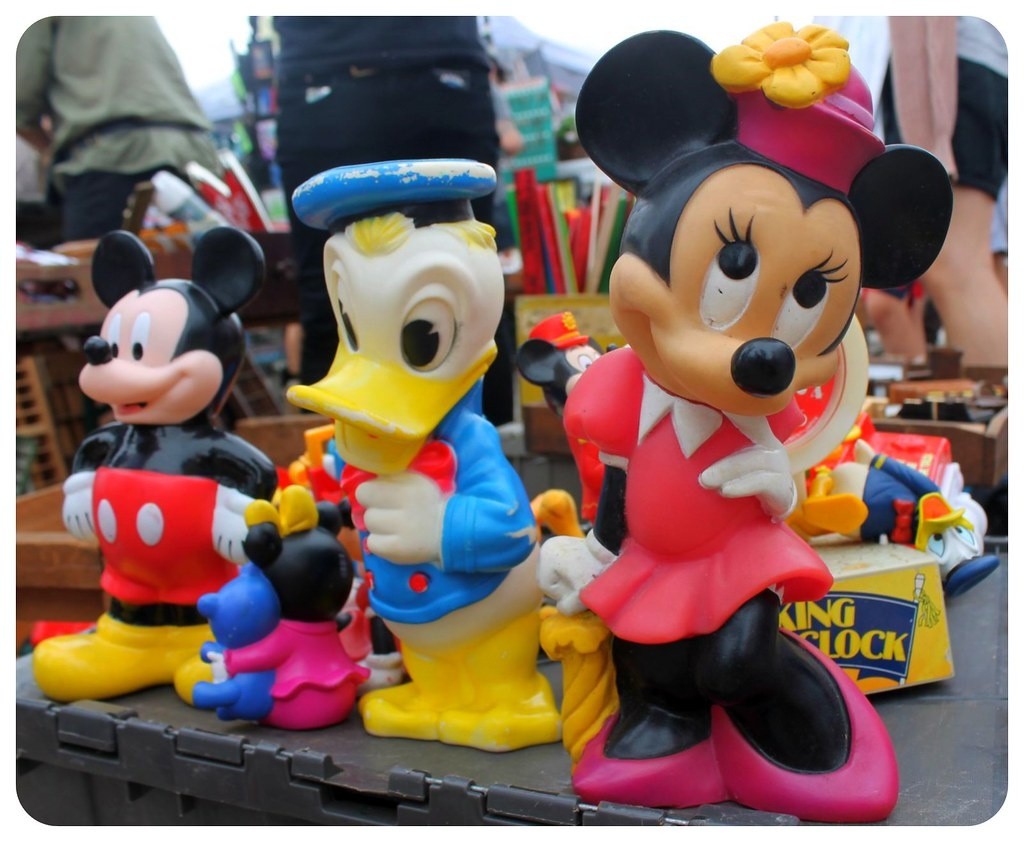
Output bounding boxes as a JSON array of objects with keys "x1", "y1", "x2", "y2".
[
  {"x1": 777, "y1": 532, "x2": 956, "y2": 696},
  {"x1": 15, "y1": 551, "x2": 1008, "y2": 826}
]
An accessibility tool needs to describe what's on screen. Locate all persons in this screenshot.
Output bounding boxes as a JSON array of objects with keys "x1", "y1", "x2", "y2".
[
  {"x1": 812, "y1": 16, "x2": 1008, "y2": 364},
  {"x1": 273, "y1": 15, "x2": 515, "y2": 430},
  {"x1": 16, "y1": 16, "x2": 220, "y2": 346}
]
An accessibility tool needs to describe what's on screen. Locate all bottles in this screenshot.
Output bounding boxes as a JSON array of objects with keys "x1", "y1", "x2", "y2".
[{"x1": 152, "y1": 172, "x2": 231, "y2": 238}]
